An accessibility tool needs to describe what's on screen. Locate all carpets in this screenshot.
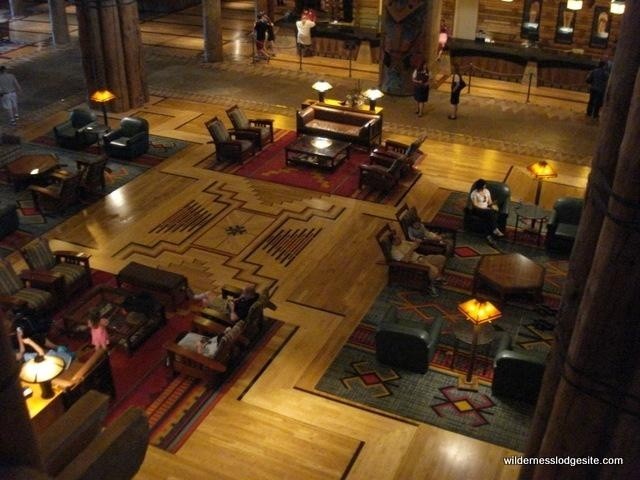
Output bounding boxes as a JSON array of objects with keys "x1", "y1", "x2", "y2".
[
  {"x1": 47, "y1": 267, "x2": 285, "y2": 453},
  {"x1": 0, "y1": 119, "x2": 190, "y2": 261},
  {"x1": 314, "y1": 190, "x2": 572, "y2": 453},
  {"x1": 202, "y1": 127, "x2": 427, "y2": 204}
]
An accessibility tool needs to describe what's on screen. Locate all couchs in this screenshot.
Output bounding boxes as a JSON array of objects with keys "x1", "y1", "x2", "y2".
[
  {"x1": 462, "y1": 179, "x2": 511, "y2": 234},
  {"x1": 544, "y1": 196, "x2": 585, "y2": 252},
  {"x1": 201, "y1": 283, "x2": 278, "y2": 339},
  {"x1": 358, "y1": 152, "x2": 412, "y2": 198},
  {"x1": 26, "y1": 171, "x2": 85, "y2": 215},
  {"x1": 57, "y1": 153, "x2": 113, "y2": 198},
  {"x1": 225, "y1": 104, "x2": 275, "y2": 151},
  {"x1": 0, "y1": 202, "x2": 19, "y2": 240},
  {"x1": 204, "y1": 115, "x2": 259, "y2": 164},
  {"x1": 491, "y1": 332, "x2": 549, "y2": 405},
  {"x1": 375, "y1": 306, "x2": 443, "y2": 375},
  {"x1": 0, "y1": 258, "x2": 66, "y2": 321},
  {"x1": 51, "y1": 347, "x2": 114, "y2": 411},
  {"x1": 395, "y1": 202, "x2": 457, "y2": 257},
  {"x1": 161, "y1": 314, "x2": 250, "y2": 386},
  {"x1": 373, "y1": 134, "x2": 429, "y2": 173},
  {"x1": 103, "y1": 116, "x2": 149, "y2": 163},
  {"x1": 52, "y1": 106, "x2": 99, "y2": 151},
  {"x1": 374, "y1": 222, "x2": 445, "y2": 293},
  {"x1": 19, "y1": 236, "x2": 93, "y2": 301},
  {"x1": 296, "y1": 105, "x2": 382, "y2": 154}
]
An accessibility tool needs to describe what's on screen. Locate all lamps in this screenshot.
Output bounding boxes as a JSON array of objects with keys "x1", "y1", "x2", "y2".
[
  {"x1": 362, "y1": 86, "x2": 385, "y2": 111},
  {"x1": 525, "y1": 160, "x2": 558, "y2": 235},
  {"x1": 609, "y1": 0, "x2": 626, "y2": 15},
  {"x1": 19, "y1": 352, "x2": 66, "y2": 399},
  {"x1": 456, "y1": 292, "x2": 503, "y2": 392},
  {"x1": 565, "y1": 1, "x2": 583, "y2": 11},
  {"x1": 312, "y1": 79, "x2": 333, "y2": 102},
  {"x1": 89, "y1": 87, "x2": 118, "y2": 134}
]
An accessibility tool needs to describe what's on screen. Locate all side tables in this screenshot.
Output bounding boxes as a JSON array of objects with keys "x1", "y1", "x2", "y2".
[
  {"x1": 513, "y1": 205, "x2": 548, "y2": 246},
  {"x1": 452, "y1": 322, "x2": 498, "y2": 375},
  {"x1": 84, "y1": 123, "x2": 111, "y2": 150}
]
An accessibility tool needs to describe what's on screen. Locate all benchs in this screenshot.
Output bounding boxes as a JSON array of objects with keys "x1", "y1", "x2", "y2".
[{"x1": 114, "y1": 260, "x2": 190, "y2": 311}]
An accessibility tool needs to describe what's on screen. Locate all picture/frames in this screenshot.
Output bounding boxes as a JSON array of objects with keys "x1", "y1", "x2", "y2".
[
  {"x1": 520, "y1": 0, "x2": 542, "y2": 41},
  {"x1": 553, "y1": 1, "x2": 577, "y2": 45},
  {"x1": 589, "y1": 5, "x2": 612, "y2": 49}
]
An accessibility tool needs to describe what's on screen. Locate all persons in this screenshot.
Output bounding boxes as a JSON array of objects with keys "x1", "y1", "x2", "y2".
[
  {"x1": 411, "y1": 59, "x2": 431, "y2": 118},
  {"x1": 389, "y1": 229, "x2": 448, "y2": 299},
  {"x1": 470, "y1": 178, "x2": 505, "y2": 247},
  {"x1": 0, "y1": 315, "x2": 58, "y2": 361},
  {"x1": 437, "y1": 26, "x2": 447, "y2": 61},
  {"x1": 296, "y1": 13, "x2": 316, "y2": 57},
  {"x1": 584, "y1": 60, "x2": 610, "y2": 120},
  {"x1": 0, "y1": 65, "x2": 25, "y2": 127},
  {"x1": 165, "y1": 330, "x2": 227, "y2": 368},
  {"x1": 251, "y1": 14, "x2": 271, "y2": 64},
  {"x1": 87, "y1": 310, "x2": 110, "y2": 353},
  {"x1": 408, "y1": 214, "x2": 444, "y2": 248},
  {"x1": 447, "y1": 60, "x2": 466, "y2": 121},
  {"x1": 226, "y1": 284, "x2": 257, "y2": 322},
  {"x1": 263, "y1": 14, "x2": 276, "y2": 56}
]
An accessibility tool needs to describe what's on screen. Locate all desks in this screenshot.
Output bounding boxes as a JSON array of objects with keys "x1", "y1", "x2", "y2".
[
  {"x1": 301, "y1": 98, "x2": 383, "y2": 115},
  {"x1": 5, "y1": 152, "x2": 60, "y2": 194},
  {"x1": 59, "y1": 283, "x2": 167, "y2": 358},
  {"x1": 472, "y1": 251, "x2": 546, "y2": 311}
]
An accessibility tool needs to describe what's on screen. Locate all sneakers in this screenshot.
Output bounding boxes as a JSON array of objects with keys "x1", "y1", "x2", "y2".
[
  {"x1": 493, "y1": 229, "x2": 504, "y2": 237},
  {"x1": 486, "y1": 236, "x2": 493, "y2": 242},
  {"x1": 435, "y1": 278, "x2": 449, "y2": 286},
  {"x1": 426, "y1": 286, "x2": 439, "y2": 298}
]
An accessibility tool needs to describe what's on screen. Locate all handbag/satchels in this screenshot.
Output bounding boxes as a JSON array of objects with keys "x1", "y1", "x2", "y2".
[{"x1": 453, "y1": 74, "x2": 466, "y2": 90}]
